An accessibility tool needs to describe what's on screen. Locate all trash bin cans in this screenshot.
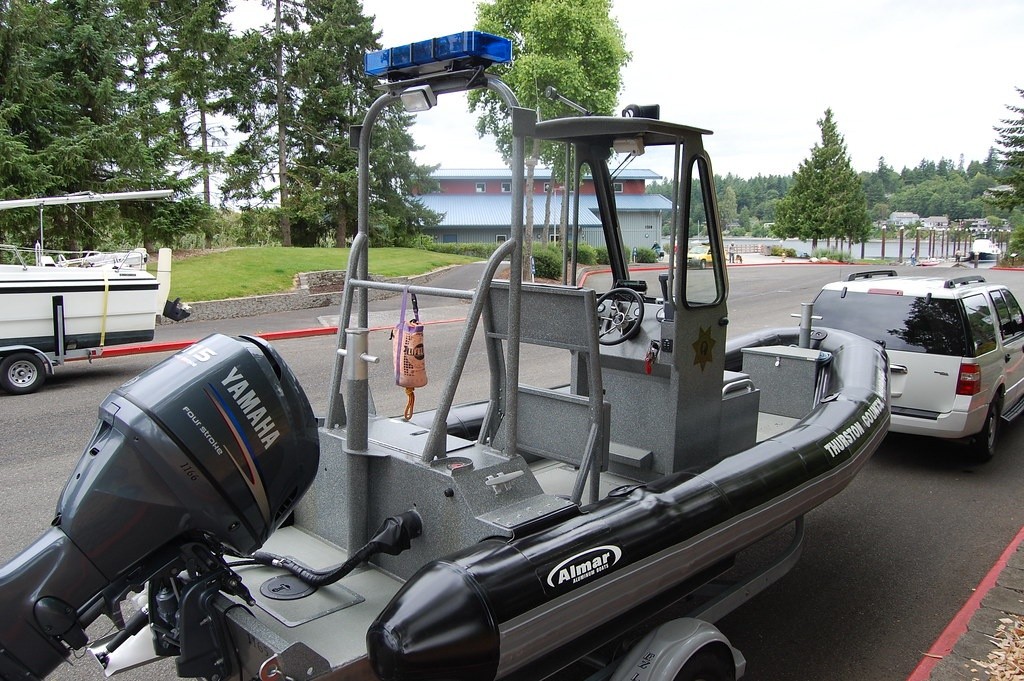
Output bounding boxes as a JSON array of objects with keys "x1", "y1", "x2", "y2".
[{"x1": 764, "y1": 246, "x2": 771, "y2": 256}]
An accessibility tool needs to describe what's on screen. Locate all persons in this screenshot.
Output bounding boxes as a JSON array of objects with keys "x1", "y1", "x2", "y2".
[
  {"x1": 910, "y1": 248, "x2": 915, "y2": 266},
  {"x1": 728, "y1": 243, "x2": 735, "y2": 263},
  {"x1": 955, "y1": 249, "x2": 961, "y2": 262},
  {"x1": 651, "y1": 241, "x2": 661, "y2": 250}
]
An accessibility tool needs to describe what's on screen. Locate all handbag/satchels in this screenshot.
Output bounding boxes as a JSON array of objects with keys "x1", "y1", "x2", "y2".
[{"x1": 389, "y1": 286, "x2": 427, "y2": 388}]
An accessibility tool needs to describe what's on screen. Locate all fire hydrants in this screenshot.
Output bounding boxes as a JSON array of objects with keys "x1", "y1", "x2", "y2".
[{"x1": 781, "y1": 252, "x2": 787, "y2": 262}]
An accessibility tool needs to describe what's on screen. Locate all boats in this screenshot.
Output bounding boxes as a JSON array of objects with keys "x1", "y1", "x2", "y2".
[
  {"x1": 0, "y1": 189, "x2": 195, "y2": 350},
  {"x1": 0, "y1": 29, "x2": 896, "y2": 681},
  {"x1": 957, "y1": 238, "x2": 1005, "y2": 264}
]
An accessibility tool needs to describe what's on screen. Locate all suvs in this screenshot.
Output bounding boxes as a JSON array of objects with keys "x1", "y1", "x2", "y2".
[{"x1": 809, "y1": 268, "x2": 1024, "y2": 466}]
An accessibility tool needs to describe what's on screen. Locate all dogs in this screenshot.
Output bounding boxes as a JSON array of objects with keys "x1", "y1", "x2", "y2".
[{"x1": 735, "y1": 255, "x2": 742, "y2": 264}]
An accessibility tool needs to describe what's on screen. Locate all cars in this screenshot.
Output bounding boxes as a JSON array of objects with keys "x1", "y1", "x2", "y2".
[{"x1": 687, "y1": 242, "x2": 728, "y2": 270}]
